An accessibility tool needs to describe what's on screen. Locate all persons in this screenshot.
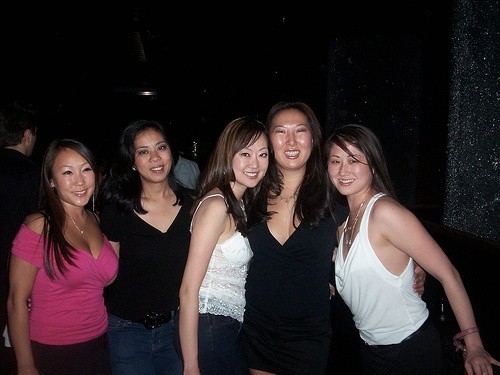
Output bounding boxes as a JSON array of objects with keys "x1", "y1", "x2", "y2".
[
  {"x1": 27, "y1": 119, "x2": 201, "y2": 375},
  {"x1": 326, "y1": 123, "x2": 500, "y2": 375},
  {"x1": 243, "y1": 100, "x2": 428, "y2": 375},
  {"x1": 178, "y1": 120, "x2": 336, "y2": 375},
  {"x1": 0, "y1": 106, "x2": 42, "y2": 375},
  {"x1": 7, "y1": 138, "x2": 118, "y2": 375},
  {"x1": 84, "y1": 134, "x2": 202, "y2": 214}
]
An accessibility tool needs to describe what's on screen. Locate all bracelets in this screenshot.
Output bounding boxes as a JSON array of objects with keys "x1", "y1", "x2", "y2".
[{"x1": 453, "y1": 327, "x2": 479, "y2": 357}]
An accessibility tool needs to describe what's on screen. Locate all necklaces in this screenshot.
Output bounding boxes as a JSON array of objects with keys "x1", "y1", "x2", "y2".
[
  {"x1": 344, "y1": 190, "x2": 372, "y2": 245},
  {"x1": 68, "y1": 213, "x2": 83, "y2": 233},
  {"x1": 240, "y1": 199, "x2": 247, "y2": 221},
  {"x1": 274, "y1": 191, "x2": 298, "y2": 205}
]
